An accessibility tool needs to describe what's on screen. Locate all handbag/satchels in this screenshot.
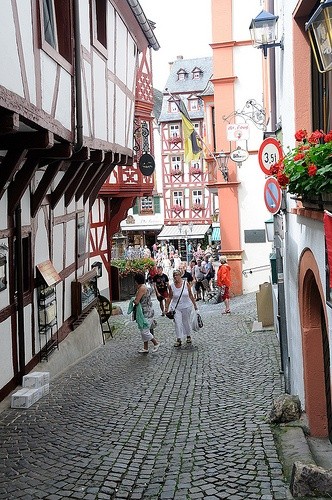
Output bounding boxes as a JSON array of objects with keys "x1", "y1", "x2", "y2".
[
  {"x1": 165, "y1": 311, "x2": 175, "y2": 319},
  {"x1": 197, "y1": 314, "x2": 204, "y2": 327},
  {"x1": 201, "y1": 279, "x2": 208, "y2": 288},
  {"x1": 130, "y1": 311, "x2": 136, "y2": 321},
  {"x1": 209, "y1": 266, "x2": 215, "y2": 278}
]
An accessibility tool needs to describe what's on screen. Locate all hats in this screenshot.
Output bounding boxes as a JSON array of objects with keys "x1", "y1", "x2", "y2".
[{"x1": 219, "y1": 256, "x2": 227, "y2": 263}]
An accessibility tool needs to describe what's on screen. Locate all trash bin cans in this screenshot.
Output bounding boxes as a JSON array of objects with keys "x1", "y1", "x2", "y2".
[{"x1": 254, "y1": 282, "x2": 274, "y2": 327}]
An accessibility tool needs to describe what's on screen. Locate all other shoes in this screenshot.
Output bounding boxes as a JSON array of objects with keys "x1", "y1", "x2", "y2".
[
  {"x1": 187, "y1": 337, "x2": 191, "y2": 343},
  {"x1": 174, "y1": 341, "x2": 181, "y2": 347},
  {"x1": 138, "y1": 349, "x2": 149, "y2": 352},
  {"x1": 152, "y1": 342, "x2": 160, "y2": 352}
]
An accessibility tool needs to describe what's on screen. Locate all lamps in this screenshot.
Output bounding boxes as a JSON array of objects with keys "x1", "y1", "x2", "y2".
[{"x1": 247, "y1": 7, "x2": 286, "y2": 59}]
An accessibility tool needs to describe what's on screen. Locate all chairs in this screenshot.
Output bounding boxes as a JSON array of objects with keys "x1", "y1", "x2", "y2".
[{"x1": 201, "y1": 279, "x2": 218, "y2": 304}]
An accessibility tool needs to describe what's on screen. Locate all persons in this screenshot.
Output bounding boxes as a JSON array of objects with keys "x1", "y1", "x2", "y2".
[
  {"x1": 217, "y1": 256, "x2": 232, "y2": 314},
  {"x1": 153, "y1": 266, "x2": 171, "y2": 316},
  {"x1": 165, "y1": 270, "x2": 199, "y2": 347},
  {"x1": 134, "y1": 273, "x2": 160, "y2": 353},
  {"x1": 126, "y1": 240, "x2": 221, "y2": 304}
]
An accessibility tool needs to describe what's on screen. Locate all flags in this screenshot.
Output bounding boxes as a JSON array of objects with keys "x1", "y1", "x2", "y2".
[{"x1": 177, "y1": 98, "x2": 207, "y2": 163}]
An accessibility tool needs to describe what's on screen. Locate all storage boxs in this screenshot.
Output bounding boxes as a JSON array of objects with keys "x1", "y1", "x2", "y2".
[{"x1": 9, "y1": 371, "x2": 51, "y2": 409}]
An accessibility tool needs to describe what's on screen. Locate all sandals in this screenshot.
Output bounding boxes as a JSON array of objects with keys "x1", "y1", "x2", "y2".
[{"x1": 222, "y1": 308, "x2": 231, "y2": 314}]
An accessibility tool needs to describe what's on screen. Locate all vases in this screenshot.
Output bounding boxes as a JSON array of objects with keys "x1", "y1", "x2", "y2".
[
  {"x1": 301, "y1": 192, "x2": 322, "y2": 209},
  {"x1": 321, "y1": 193, "x2": 332, "y2": 212}
]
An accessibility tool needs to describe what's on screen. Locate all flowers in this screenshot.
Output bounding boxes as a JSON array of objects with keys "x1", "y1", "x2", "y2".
[{"x1": 266, "y1": 125, "x2": 332, "y2": 197}]
